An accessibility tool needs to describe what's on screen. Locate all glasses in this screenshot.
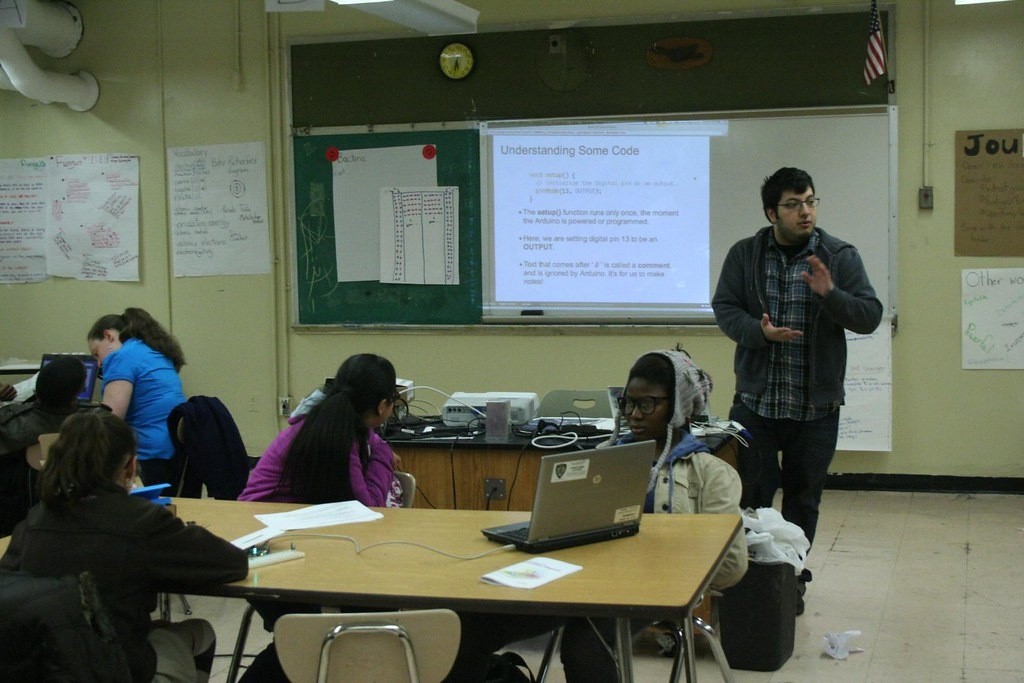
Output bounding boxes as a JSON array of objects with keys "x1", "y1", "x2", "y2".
[
  {"x1": 617, "y1": 396, "x2": 670, "y2": 414},
  {"x1": 772, "y1": 198, "x2": 819, "y2": 209},
  {"x1": 380, "y1": 392, "x2": 399, "y2": 401}
]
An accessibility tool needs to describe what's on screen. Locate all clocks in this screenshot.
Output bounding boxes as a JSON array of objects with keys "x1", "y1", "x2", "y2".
[{"x1": 438, "y1": 42, "x2": 476, "y2": 83}]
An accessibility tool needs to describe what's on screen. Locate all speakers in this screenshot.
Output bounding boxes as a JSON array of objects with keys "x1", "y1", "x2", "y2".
[{"x1": 484, "y1": 398, "x2": 512, "y2": 442}]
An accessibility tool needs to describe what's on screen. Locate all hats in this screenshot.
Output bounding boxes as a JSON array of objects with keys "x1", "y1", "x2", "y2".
[{"x1": 595, "y1": 351, "x2": 710, "y2": 493}]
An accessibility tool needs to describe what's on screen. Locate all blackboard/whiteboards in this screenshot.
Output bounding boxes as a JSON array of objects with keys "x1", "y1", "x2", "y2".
[{"x1": 287, "y1": 101, "x2": 900, "y2": 334}]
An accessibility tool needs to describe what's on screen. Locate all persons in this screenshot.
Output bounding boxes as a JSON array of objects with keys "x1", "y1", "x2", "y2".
[
  {"x1": 460, "y1": 346, "x2": 751, "y2": 683},
  {"x1": 0, "y1": 356, "x2": 87, "y2": 537},
  {"x1": 86, "y1": 307, "x2": 187, "y2": 486},
  {"x1": 0, "y1": 406, "x2": 248, "y2": 683},
  {"x1": 234, "y1": 355, "x2": 406, "y2": 683},
  {"x1": 711, "y1": 166, "x2": 883, "y2": 613}
]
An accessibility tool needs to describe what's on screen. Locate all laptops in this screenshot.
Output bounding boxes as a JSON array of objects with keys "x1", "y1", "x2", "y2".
[
  {"x1": 480, "y1": 439, "x2": 658, "y2": 554},
  {"x1": 39, "y1": 352, "x2": 101, "y2": 408}
]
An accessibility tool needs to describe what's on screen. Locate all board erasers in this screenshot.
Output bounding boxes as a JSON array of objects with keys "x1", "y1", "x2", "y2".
[{"x1": 520, "y1": 310, "x2": 544, "y2": 315}]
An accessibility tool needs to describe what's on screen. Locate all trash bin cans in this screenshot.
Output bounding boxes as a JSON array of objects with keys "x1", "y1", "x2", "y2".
[{"x1": 718, "y1": 562, "x2": 796, "y2": 672}]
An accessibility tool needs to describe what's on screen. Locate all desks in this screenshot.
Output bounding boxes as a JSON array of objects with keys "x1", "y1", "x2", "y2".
[{"x1": 0, "y1": 486, "x2": 742, "y2": 683}]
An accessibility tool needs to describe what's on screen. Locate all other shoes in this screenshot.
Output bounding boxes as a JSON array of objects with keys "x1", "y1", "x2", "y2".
[{"x1": 796, "y1": 588, "x2": 804, "y2": 615}]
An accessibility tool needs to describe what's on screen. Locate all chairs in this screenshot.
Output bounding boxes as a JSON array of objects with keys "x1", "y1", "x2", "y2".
[
  {"x1": 275, "y1": 609, "x2": 463, "y2": 683},
  {"x1": 166, "y1": 396, "x2": 250, "y2": 498},
  {"x1": 535, "y1": 390, "x2": 614, "y2": 418},
  {"x1": 226, "y1": 471, "x2": 418, "y2": 683}
]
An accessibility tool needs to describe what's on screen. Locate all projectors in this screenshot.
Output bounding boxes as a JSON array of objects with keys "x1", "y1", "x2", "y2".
[{"x1": 442, "y1": 391, "x2": 540, "y2": 430}]
